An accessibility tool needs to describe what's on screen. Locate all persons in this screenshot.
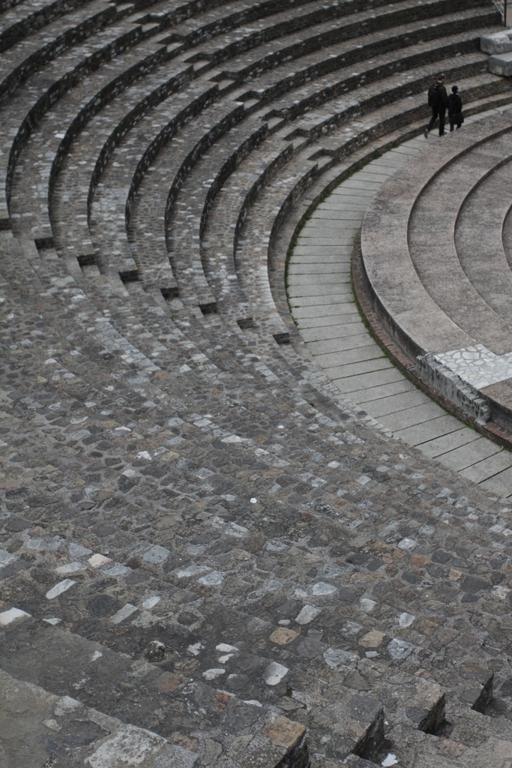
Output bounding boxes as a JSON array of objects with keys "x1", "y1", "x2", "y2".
[
  {"x1": 423, "y1": 69, "x2": 447, "y2": 136},
  {"x1": 445, "y1": 86, "x2": 465, "y2": 134}
]
